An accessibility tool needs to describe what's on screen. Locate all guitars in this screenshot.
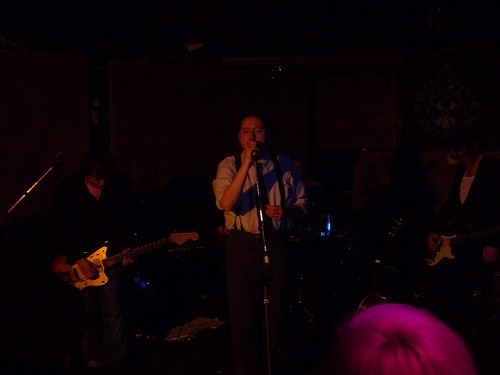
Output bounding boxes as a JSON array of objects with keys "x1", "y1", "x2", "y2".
[
  {"x1": 66, "y1": 228, "x2": 204, "y2": 292},
  {"x1": 423, "y1": 225, "x2": 497, "y2": 267}
]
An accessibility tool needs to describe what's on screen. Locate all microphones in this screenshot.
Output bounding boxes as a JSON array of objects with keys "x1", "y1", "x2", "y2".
[{"x1": 253, "y1": 150, "x2": 257, "y2": 158}]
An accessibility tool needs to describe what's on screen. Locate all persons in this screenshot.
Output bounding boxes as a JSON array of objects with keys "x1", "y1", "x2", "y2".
[
  {"x1": 319, "y1": 303, "x2": 478, "y2": 375},
  {"x1": 51, "y1": 149, "x2": 134, "y2": 375},
  {"x1": 211, "y1": 109, "x2": 310, "y2": 375},
  {"x1": 426, "y1": 130, "x2": 500, "y2": 320}
]
